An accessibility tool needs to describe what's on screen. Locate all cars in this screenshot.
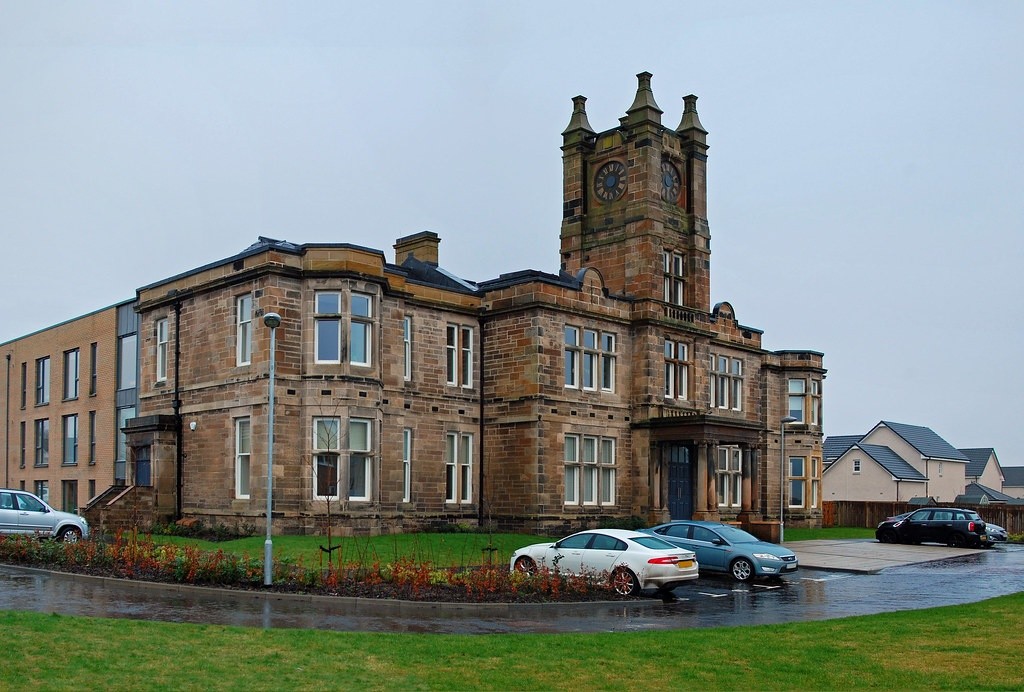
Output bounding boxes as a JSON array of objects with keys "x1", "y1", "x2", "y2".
[
  {"x1": 510, "y1": 528, "x2": 698, "y2": 596},
  {"x1": 0, "y1": 488, "x2": 89, "y2": 545},
  {"x1": 634, "y1": 521, "x2": 798, "y2": 583},
  {"x1": 886, "y1": 512, "x2": 1007, "y2": 548}
]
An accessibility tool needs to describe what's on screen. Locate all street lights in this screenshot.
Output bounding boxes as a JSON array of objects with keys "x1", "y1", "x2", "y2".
[
  {"x1": 780, "y1": 416, "x2": 797, "y2": 541},
  {"x1": 262, "y1": 312, "x2": 281, "y2": 588}
]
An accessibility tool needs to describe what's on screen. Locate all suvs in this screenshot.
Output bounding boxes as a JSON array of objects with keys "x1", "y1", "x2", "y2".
[{"x1": 875, "y1": 508, "x2": 987, "y2": 547}]
